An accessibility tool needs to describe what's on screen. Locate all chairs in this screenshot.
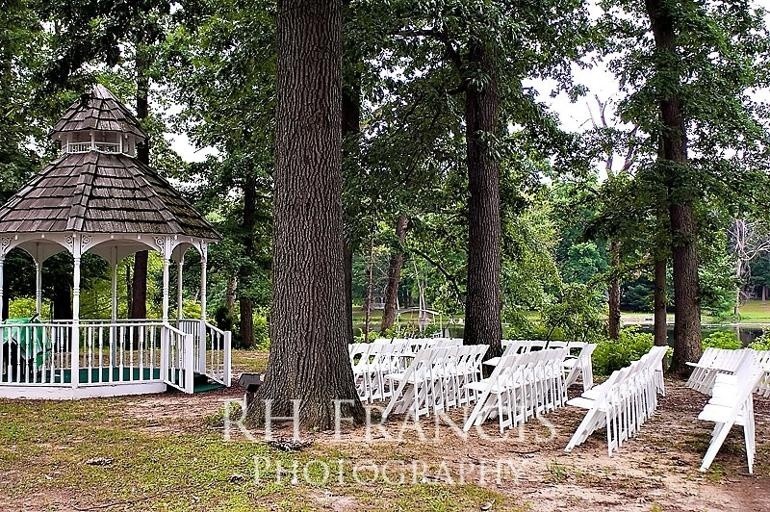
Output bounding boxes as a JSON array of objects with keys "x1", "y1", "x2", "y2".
[{"x1": 347, "y1": 337, "x2": 770, "y2": 472}]
